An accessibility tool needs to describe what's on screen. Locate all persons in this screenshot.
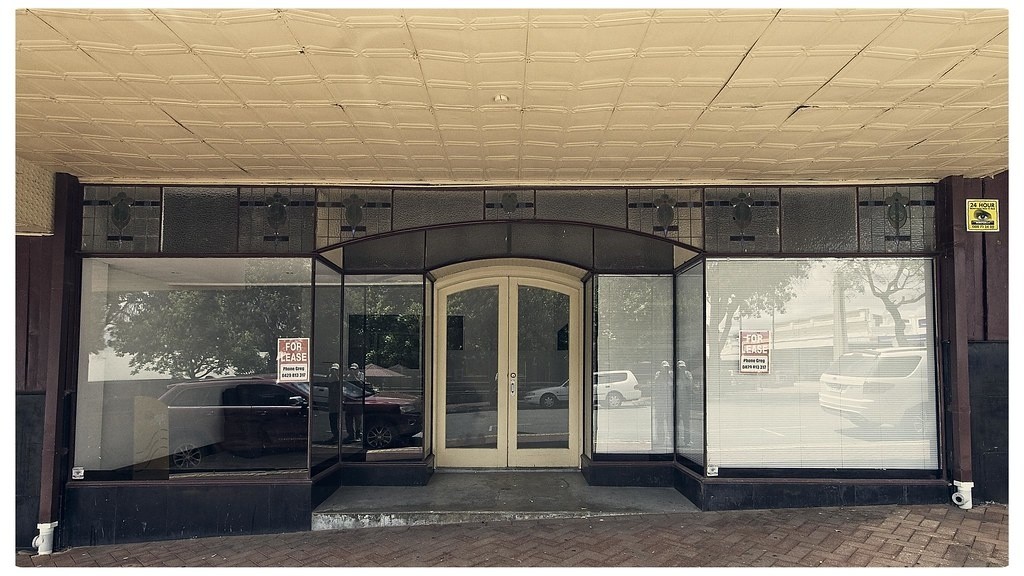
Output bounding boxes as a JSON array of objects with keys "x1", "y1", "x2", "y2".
[
  {"x1": 653, "y1": 360, "x2": 693, "y2": 447},
  {"x1": 322, "y1": 363, "x2": 364, "y2": 443}
]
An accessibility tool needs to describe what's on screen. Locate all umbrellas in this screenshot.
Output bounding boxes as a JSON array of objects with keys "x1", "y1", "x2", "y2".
[{"x1": 365, "y1": 363, "x2": 420, "y2": 397}]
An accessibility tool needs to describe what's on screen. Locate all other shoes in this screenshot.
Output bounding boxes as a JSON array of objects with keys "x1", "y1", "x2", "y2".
[
  {"x1": 355, "y1": 430, "x2": 361, "y2": 442},
  {"x1": 328, "y1": 437, "x2": 338, "y2": 442},
  {"x1": 343, "y1": 435, "x2": 353, "y2": 442}
]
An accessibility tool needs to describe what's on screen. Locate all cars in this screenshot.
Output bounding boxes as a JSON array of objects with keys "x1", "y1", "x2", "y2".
[{"x1": 312, "y1": 373, "x2": 330, "y2": 406}]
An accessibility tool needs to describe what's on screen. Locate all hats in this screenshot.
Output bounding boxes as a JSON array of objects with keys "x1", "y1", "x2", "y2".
[
  {"x1": 331, "y1": 363, "x2": 340, "y2": 370},
  {"x1": 350, "y1": 362, "x2": 358, "y2": 369}
]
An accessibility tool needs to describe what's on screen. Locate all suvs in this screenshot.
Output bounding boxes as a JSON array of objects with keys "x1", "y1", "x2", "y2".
[
  {"x1": 818, "y1": 346, "x2": 930, "y2": 438},
  {"x1": 524, "y1": 369, "x2": 641, "y2": 409},
  {"x1": 151, "y1": 375, "x2": 425, "y2": 468}
]
